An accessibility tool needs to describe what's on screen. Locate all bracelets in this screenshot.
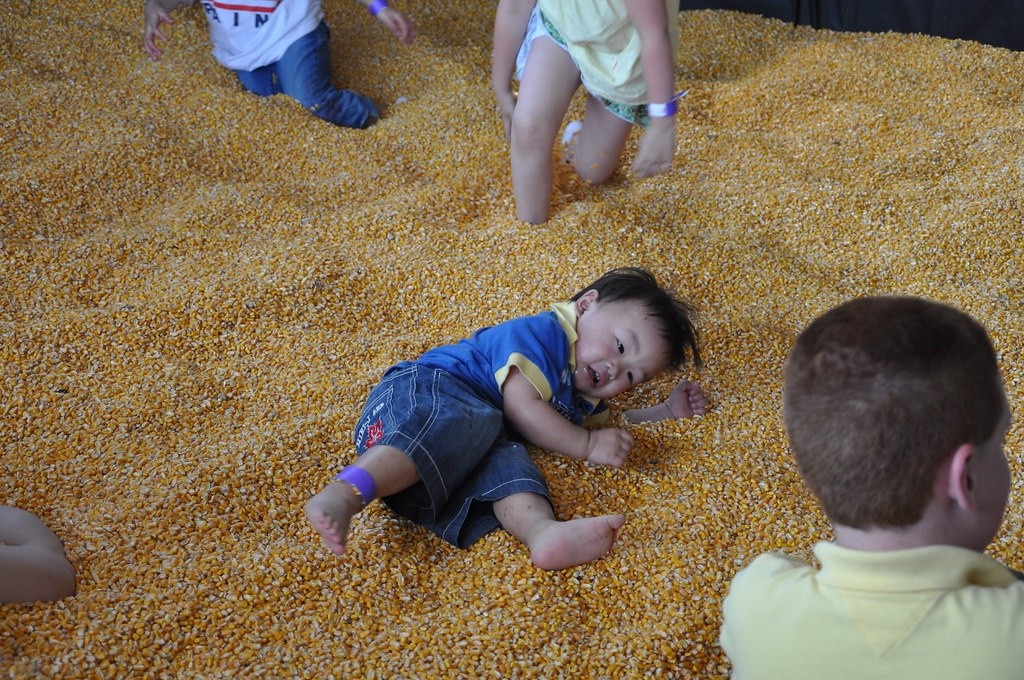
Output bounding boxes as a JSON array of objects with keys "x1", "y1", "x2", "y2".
[
  {"x1": 368, "y1": 0, "x2": 389, "y2": 16},
  {"x1": 646, "y1": 89, "x2": 690, "y2": 118}
]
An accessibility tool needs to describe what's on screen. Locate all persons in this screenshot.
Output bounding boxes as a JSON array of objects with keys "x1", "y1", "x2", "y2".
[
  {"x1": 487, "y1": 0, "x2": 680, "y2": 226},
  {"x1": 141, "y1": 0, "x2": 416, "y2": 129},
  {"x1": 303, "y1": 266, "x2": 709, "y2": 573},
  {"x1": 716, "y1": 294, "x2": 1024, "y2": 680}
]
